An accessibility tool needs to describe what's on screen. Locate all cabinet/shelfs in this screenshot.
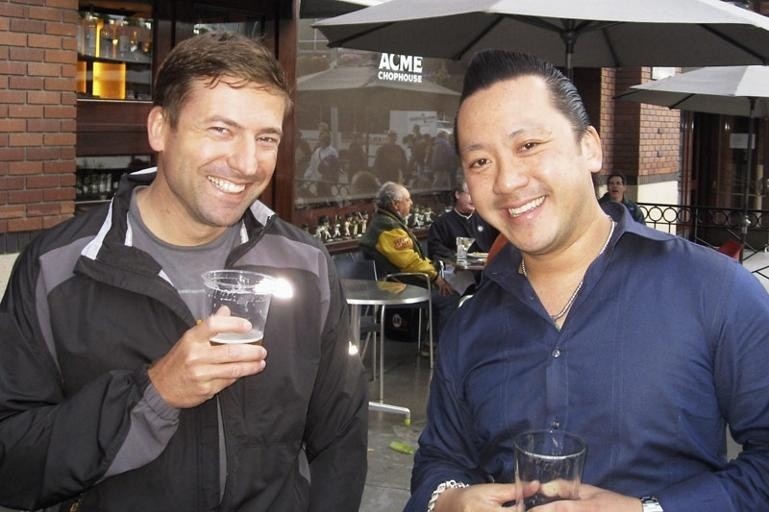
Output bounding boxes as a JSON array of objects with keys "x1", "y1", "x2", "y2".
[{"x1": 77, "y1": 6, "x2": 154, "y2": 105}]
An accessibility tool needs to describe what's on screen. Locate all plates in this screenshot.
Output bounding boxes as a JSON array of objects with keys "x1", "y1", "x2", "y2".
[{"x1": 467, "y1": 252, "x2": 490, "y2": 259}]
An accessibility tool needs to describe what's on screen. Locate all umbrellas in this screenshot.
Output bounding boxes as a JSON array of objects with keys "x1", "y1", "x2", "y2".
[
  {"x1": 291, "y1": 64, "x2": 464, "y2": 114},
  {"x1": 312, "y1": 0, "x2": 769, "y2": 90},
  {"x1": 612, "y1": 65, "x2": 769, "y2": 267}
]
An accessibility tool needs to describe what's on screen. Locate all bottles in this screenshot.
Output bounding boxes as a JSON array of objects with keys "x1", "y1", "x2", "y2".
[
  {"x1": 75, "y1": 159, "x2": 111, "y2": 199},
  {"x1": 77, "y1": 9, "x2": 154, "y2": 62}
]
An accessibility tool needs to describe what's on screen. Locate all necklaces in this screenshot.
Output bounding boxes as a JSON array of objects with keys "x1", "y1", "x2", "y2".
[{"x1": 518, "y1": 220, "x2": 618, "y2": 322}]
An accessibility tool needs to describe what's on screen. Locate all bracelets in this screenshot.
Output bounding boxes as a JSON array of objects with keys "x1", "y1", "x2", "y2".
[{"x1": 427, "y1": 479, "x2": 471, "y2": 510}]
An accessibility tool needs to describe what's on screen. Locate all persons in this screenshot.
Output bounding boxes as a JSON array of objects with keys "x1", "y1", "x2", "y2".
[
  {"x1": 403, "y1": 46, "x2": 769, "y2": 510},
  {"x1": 358, "y1": 182, "x2": 462, "y2": 361},
  {"x1": 429, "y1": 176, "x2": 500, "y2": 293},
  {"x1": 597, "y1": 170, "x2": 645, "y2": 227},
  {"x1": 289, "y1": 120, "x2": 463, "y2": 205},
  {"x1": 0, "y1": 26, "x2": 369, "y2": 512}
]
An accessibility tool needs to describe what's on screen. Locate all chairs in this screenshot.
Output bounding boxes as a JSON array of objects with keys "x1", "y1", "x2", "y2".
[
  {"x1": 360, "y1": 242, "x2": 436, "y2": 371},
  {"x1": 718, "y1": 241, "x2": 743, "y2": 262},
  {"x1": 330, "y1": 254, "x2": 379, "y2": 384}
]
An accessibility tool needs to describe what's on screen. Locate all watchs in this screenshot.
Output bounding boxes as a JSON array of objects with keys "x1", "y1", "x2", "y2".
[{"x1": 639, "y1": 494, "x2": 665, "y2": 511}]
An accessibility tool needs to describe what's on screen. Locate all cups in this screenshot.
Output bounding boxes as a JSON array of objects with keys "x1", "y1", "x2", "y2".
[
  {"x1": 513, "y1": 429, "x2": 588, "y2": 512},
  {"x1": 456, "y1": 236, "x2": 476, "y2": 263},
  {"x1": 199, "y1": 269, "x2": 274, "y2": 356}
]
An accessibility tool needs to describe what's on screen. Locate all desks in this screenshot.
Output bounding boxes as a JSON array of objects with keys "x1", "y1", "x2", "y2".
[
  {"x1": 453, "y1": 247, "x2": 490, "y2": 286},
  {"x1": 343, "y1": 276, "x2": 434, "y2": 426}
]
